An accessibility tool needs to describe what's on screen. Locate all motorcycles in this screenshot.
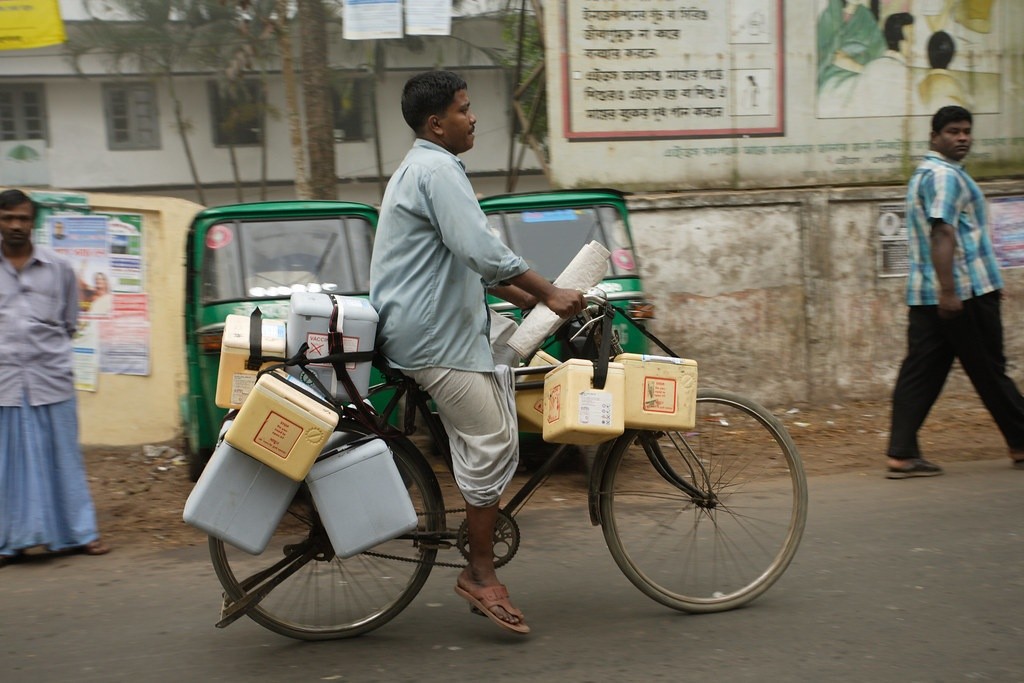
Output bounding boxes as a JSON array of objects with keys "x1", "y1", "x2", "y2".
[
  {"x1": 177, "y1": 198, "x2": 416, "y2": 481},
  {"x1": 469, "y1": 183, "x2": 658, "y2": 474}
]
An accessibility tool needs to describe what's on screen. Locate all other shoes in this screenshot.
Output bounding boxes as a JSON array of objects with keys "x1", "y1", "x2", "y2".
[
  {"x1": 83, "y1": 540, "x2": 112, "y2": 556},
  {"x1": 3, "y1": 550, "x2": 23, "y2": 564}
]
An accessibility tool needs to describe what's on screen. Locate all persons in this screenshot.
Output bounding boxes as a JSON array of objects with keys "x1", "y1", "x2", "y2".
[
  {"x1": 90, "y1": 272, "x2": 108, "y2": 299},
  {"x1": 370, "y1": 70, "x2": 588, "y2": 634},
  {"x1": 51, "y1": 222, "x2": 66, "y2": 240},
  {"x1": 0, "y1": 189, "x2": 111, "y2": 565},
  {"x1": 885, "y1": 106, "x2": 1024, "y2": 479}
]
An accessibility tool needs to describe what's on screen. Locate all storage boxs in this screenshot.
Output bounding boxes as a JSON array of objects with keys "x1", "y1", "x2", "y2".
[
  {"x1": 511, "y1": 348, "x2": 562, "y2": 438},
  {"x1": 216, "y1": 311, "x2": 289, "y2": 410},
  {"x1": 539, "y1": 357, "x2": 626, "y2": 448},
  {"x1": 614, "y1": 354, "x2": 700, "y2": 430},
  {"x1": 285, "y1": 290, "x2": 381, "y2": 409},
  {"x1": 301, "y1": 436, "x2": 419, "y2": 560},
  {"x1": 181, "y1": 420, "x2": 301, "y2": 557},
  {"x1": 225, "y1": 372, "x2": 340, "y2": 480}
]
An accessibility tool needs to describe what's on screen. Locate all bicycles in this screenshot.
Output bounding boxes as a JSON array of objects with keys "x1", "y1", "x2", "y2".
[{"x1": 203, "y1": 280, "x2": 809, "y2": 643}]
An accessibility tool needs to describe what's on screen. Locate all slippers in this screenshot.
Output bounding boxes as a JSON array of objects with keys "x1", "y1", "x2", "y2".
[
  {"x1": 454, "y1": 582, "x2": 530, "y2": 634},
  {"x1": 1011, "y1": 460, "x2": 1023, "y2": 470},
  {"x1": 885, "y1": 458, "x2": 944, "y2": 478}
]
